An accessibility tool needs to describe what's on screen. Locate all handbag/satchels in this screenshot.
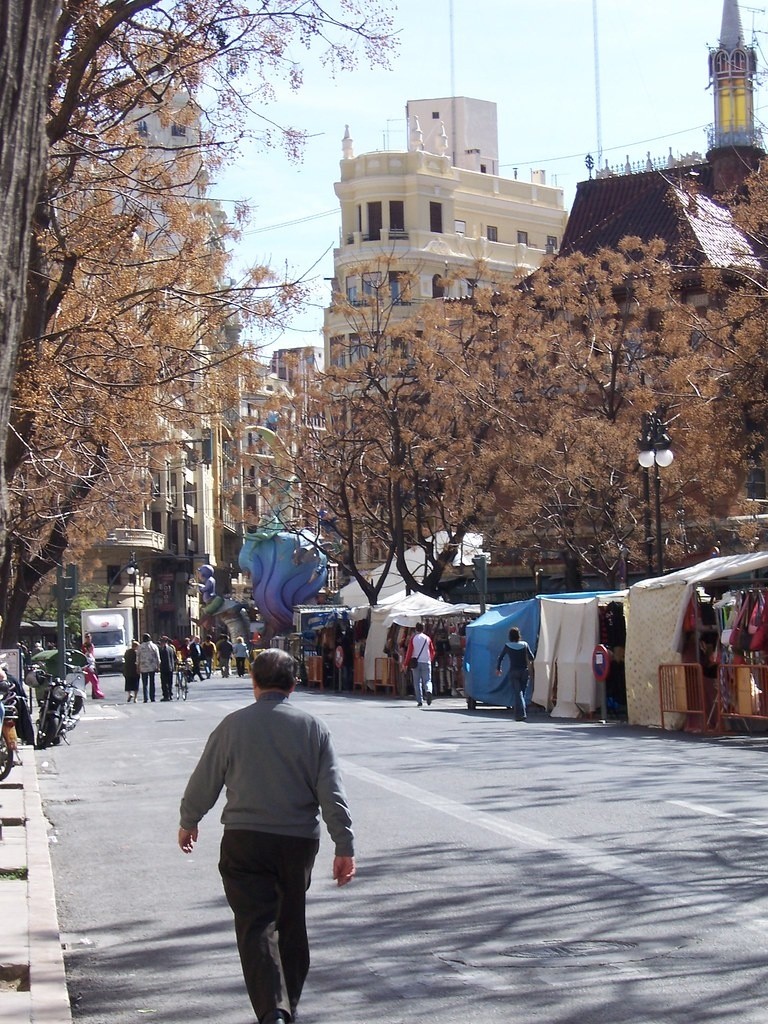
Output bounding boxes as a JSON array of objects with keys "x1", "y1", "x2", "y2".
[
  {"x1": 410, "y1": 658, "x2": 417, "y2": 668},
  {"x1": 685, "y1": 584, "x2": 767, "y2": 652},
  {"x1": 425, "y1": 617, "x2": 472, "y2": 650}
]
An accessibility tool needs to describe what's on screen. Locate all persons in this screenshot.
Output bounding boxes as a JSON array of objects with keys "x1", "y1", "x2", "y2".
[
  {"x1": 155, "y1": 634, "x2": 251, "y2": 681},
  {"x1": 177, "y1": 649, "x2": 356, "y2": 1024},
  {"x1": 496, "y1": 626, "x2": 534, "y2": 720},
  {"x1": 399, "y1": 622, "x2": 435, "y2": 706},
  {"x1": 14, "y1": 633, "x2": 108, "y2": 699},
  {"x1": 190, "y1": 564, "x2": 217, "y2": 605},
  {"x1": 123, "y1": 633, "x2": 177, "y2": 703}
]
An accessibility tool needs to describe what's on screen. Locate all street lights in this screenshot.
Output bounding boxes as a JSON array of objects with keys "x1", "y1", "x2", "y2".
[
  {"x1": 636, "y1": 401, "x2": 674, "y2": 579},
  {"x1": 126, "y1": 555, "x2": 139, "y2": 641}
]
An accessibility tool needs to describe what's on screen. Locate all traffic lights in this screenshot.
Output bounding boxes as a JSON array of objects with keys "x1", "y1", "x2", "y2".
[
  {"x1": 56, "y1": 577, "x2": 73, "y2": 612},
  {"x1": 472, "y1": 558, "x2": 483, "y2": 594}
]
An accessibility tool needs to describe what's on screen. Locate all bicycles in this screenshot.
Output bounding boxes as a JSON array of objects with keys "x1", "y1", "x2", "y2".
[{"x1": 174, "y1": 660, "x2": 193, "y2": 701}]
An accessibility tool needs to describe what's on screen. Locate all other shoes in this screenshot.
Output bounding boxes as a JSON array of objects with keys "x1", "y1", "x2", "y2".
[
  {"x1": 151, "y1": 699, "x2": 155, "y2": 702},
  {"x1": 516, "y1": 716, "x2": 528, "y2": 721},
  {"x1": 134, "y1": 699, "x2": 137, "y2": 703},
  {"x1": 143, "y1": 700, "x2": 147, "y2": 703},
  {"x1": 201, "y1": 678, "x2": 205, "y2": 681},
  {"x1": 418, "y1": 702, "x2": 422, "y2": 706},
  {"x1": 427, "y1": 692, "x2": 431, "y2": 705},
  {"x1": 161, "y1": 698, "x2": 170, "y2": 701},
  {"x1": 262, "y1": 1010, "x2": 285, "y2": 1024},
  {"x1": 127, "y1": 694, "x2": 133, "y2": 702}
]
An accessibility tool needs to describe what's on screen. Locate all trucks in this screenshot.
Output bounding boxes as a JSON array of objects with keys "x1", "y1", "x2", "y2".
[{"x1": 81, "y1": 607, "x2": 133, "y2": 675}]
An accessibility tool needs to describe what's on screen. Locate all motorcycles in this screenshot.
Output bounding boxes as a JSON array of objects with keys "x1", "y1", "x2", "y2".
[{"x1": 32, "y1": 671, "x2": 86, "y2": 748}]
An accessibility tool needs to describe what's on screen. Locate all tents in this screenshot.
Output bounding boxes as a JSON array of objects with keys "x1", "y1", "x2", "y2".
[{"x1": 347, "y1": 550, "x2": 768, "y2": 728}]
